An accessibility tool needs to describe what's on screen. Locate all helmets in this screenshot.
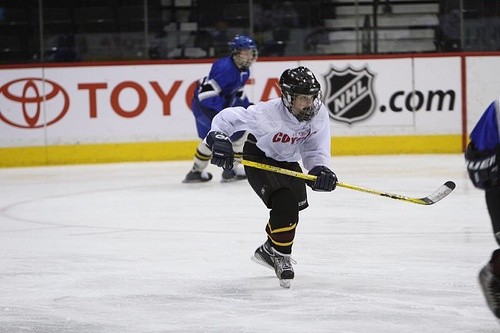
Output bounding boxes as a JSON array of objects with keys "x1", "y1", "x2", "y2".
[
  {"x1": 228, "y1": 35, "x2": 258, "y2": 66},
  {"x1": 279, "y1": 66, "x2": 322, "y2": 119}
]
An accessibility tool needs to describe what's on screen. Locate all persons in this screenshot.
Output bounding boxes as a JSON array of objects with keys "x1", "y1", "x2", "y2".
[
  {"x1": 51, "y1": 17, "x2": 77, "y2": 62},
  {"x1": 181, "y1": 35, "x2": 258, "y2": 183},
  {"x1": 202, "y1": 66, "x2": 338, "y2": 288},
  {"x1": 464, "y1": 100, "x2": 500, "y2": 319},
  {"x1": 147, "y1": 16, "x2": 291, "y2": 59}
]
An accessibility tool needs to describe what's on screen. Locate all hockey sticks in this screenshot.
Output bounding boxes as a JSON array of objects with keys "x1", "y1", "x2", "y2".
[{"x1": 232, "y1": 156, "x2": 457, "y2": 205}]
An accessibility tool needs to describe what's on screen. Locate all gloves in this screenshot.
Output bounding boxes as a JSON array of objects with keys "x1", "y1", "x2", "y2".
[
  {"x1": 304, "y1": 166, "x2": 337, "y2": 192},
  {"x1": 205, "y1": 130, "x2": 234, "y2": 172}
]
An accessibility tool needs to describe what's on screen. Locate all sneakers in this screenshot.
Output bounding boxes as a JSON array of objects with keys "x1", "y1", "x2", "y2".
[
  {"x1": 186, "y1": 169, "x2": 212, "y2": 183},
  {"x1": 479, "y1": 263, "x2": 500, "y2": 318},
  {"x1": 221, "y1": 170, "x2": 246, "y2": 180},
  {"x1": 273, "y1": 253, "x2": 295, "y2": 287},
  {"x1": 251, "y1": 241, "x2": 274, "y2": 267}
]
